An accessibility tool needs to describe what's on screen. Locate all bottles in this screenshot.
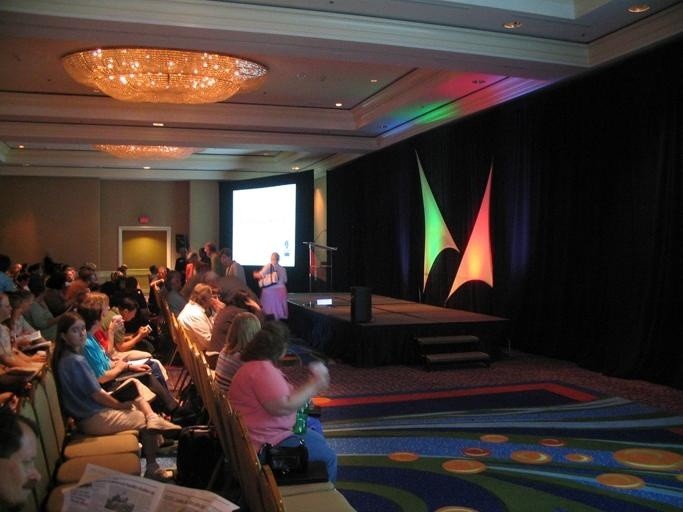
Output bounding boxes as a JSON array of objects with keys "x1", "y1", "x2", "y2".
[{"x1": 294, "y1": 400, "x2": 309, "y2": 435}]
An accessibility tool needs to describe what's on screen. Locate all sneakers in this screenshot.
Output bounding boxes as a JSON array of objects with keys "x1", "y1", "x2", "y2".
[
  {"x1": 146, "y1": 416, "x2": 181, "y2": 433},
  {"x1": 144, "y1": 469, "x2": 168, "y2": 482}
]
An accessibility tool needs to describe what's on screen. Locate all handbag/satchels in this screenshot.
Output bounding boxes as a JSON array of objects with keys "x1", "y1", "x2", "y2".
[
  {"x1": 262, "y1": 440, "x2": 309, "y2": 484},
  {"x1": 257, "y1": 264, "x2": 278, "y2": 288}
]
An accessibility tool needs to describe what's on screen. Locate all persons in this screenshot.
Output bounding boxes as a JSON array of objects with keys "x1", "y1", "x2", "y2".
[
  {"x1": 1, "y1": 409, "x2": 43, "y2": 511},
  {"x1": 1, "y1": 243, "x2": 331, "y2": 482}
]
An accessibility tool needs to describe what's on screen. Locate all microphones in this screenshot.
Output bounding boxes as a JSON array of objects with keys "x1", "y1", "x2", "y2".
[{"x1": 311, "y1": 229, "x2": 330, "y2": 242}]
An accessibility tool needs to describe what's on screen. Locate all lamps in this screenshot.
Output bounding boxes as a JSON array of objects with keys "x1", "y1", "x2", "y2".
[
  {"x1": 92, "y1": 143, "x2": 201, "y2": 162},
  {"x1": 59, "y1": 47, "x2": 268, "y2": 104}
]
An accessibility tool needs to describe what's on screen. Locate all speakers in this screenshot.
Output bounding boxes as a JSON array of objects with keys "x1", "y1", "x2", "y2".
[{"x1": 350, "y1": 286, "x2": 373, "y2": 322}]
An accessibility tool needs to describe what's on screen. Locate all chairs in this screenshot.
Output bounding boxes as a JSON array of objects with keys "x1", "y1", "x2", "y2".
[
  {"x1": 149, "y1": 280, "x2": 197, "y2": 346},
  {"x1": 166, "y1": 346, "x2": 233, "y2": 489},
  {"x1": 258, "y1": 466, "x2": 357, "y2": 512},
  {"x1": 229, "y1": 412, "x2": 334, "y2": 508},
  {"x1": 69, "y1": 427, "x2": 140, "y2": 437},
  {"x1": 32, "y1": 383, "x2": 141, "y2": 483},
  {"x1": 20, "y1": 400, "x2": 90, "y2": 511},
  {"x1": 41, "y1": 368, "x2": 142, "y2": 458}
]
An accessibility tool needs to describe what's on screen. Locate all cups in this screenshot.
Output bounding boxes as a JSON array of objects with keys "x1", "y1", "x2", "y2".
[{"x1": 111, "y1": 315, "x2": 122, "y2": 323}]
